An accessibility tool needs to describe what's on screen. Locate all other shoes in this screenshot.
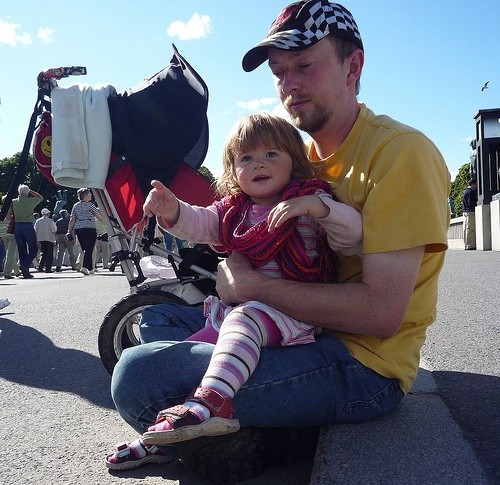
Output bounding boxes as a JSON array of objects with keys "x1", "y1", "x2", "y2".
[
  {"x1": 16, "y1": 272, "x2": 21, "y2": 277},
  {"x1": 177, "y1": 426, "x2": 319, "y2": 485},
  {"x1": 46, "y1": 269, "x2": 55, "y2": 273},
  {"x1": 94, "y1": 268, "x2": 98, "y2": 272},
  {"x1": 19, "y1": 265, "x2": 33, "y2": 278},
  {"x1": 0, "y1": 297, "x2": 11, "y2": 309},
  {"x1": 75, "y1": 263, "x2": 81, "y2": 272},
  {"x1": 5, "y1": 275, "x2": 14, "y2": 279}
]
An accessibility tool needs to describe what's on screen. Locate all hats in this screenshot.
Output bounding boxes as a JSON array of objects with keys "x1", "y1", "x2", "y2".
[
  {"x1": 242, "y1": 0, "x2": 364, "y2": 72},
  {"x1": 41, "y1": 208, "x2": 51, "y2": 214}
]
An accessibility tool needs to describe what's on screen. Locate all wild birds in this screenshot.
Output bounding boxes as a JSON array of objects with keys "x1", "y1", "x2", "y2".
[{"x1": 481, "y1": 81, "x2": 490, "y2": 92}]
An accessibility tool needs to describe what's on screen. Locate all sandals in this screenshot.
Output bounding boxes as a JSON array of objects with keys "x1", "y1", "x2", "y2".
[
  {"x1": 142, "y1": 387, "x2": 240, "y2": 444},
  {"x1": 104, "y1": 435, "x2": 173, "y2": 470}
]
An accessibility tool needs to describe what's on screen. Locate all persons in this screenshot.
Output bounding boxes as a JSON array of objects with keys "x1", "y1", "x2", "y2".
[
  {"x1": 0, "y1": 183, "x2": 110, "y2": 278},
  {"x1": 463, "y1": 177, "x2": 478, "y2": 250},
  {"x1": 110, "y1": 0, "x2": 451, "y2": 483},
  {"x1": 105, "y1": 116, "x2": 364, "y2": 472}
]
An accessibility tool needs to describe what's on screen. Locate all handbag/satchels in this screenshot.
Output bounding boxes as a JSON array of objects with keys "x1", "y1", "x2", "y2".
[{"x1": 7, "y1": 219, "x2": 15, "y2": 234}]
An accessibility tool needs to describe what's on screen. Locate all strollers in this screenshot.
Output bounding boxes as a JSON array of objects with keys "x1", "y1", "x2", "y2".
[{"x1": 36, "y1": 42, "x2": 217, "y2": 378}]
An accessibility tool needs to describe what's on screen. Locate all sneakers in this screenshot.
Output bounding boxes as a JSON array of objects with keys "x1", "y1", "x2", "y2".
[
  {"x1": 80, "y1": 267, "x2": 90, "y2": 274},
  {"x1": 90, "y1": 269, "x2": 94, "y2": 274}
]
